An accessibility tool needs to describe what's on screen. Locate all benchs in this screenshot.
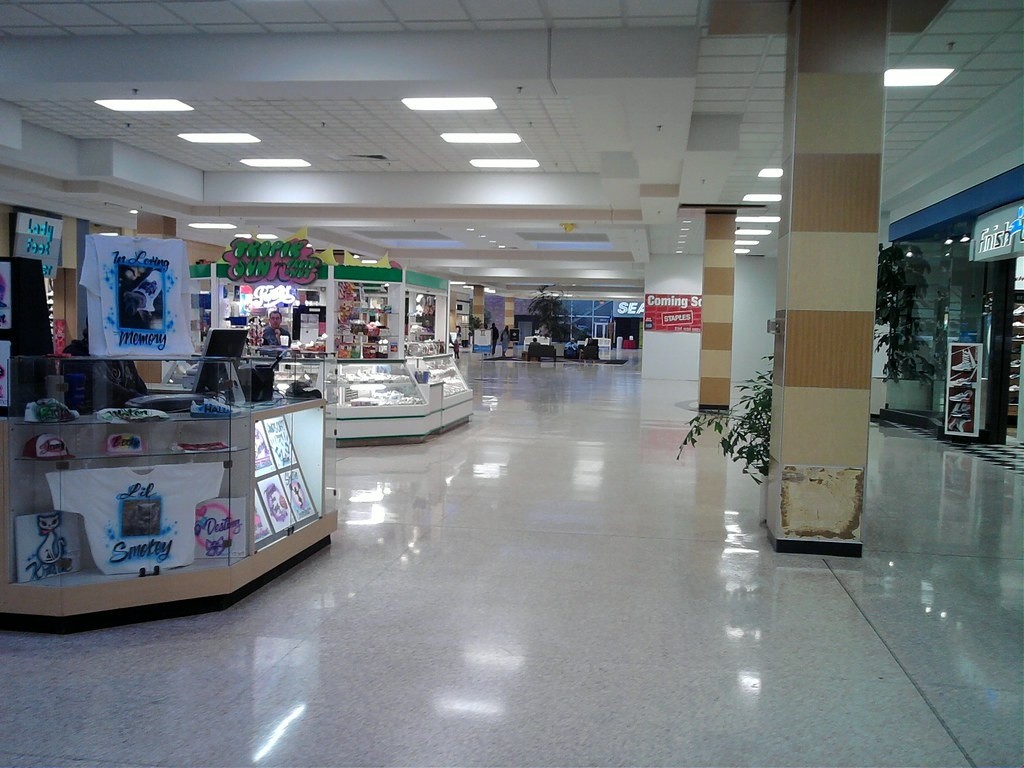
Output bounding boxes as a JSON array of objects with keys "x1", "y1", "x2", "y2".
[{"x1": 527, "y1": 345, "x2": 557, "y2": 362}]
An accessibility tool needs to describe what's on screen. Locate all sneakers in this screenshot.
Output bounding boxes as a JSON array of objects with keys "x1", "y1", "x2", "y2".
[
  {"x1": 190, "y1": 397, "x2": 242, "y2": 418},
  {"x1": 25, "y1": 397, "x2": 80, "y2": 423}
]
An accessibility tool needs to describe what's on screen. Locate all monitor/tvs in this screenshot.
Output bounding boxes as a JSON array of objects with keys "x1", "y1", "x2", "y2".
[{"x1": 192, "y1": 329, "x2": 249, "y2": 394}]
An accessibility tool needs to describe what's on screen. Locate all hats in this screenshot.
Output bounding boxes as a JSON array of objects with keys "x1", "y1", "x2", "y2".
[
  {"x1": 570, "y1": 338, "x2": 575, "y2": 341},
  {"x1": 101, "y1": 432, "x2": 149, "y2": 456},
  {"x1": 23, "y1": 433, "x2": 76, "y2": 460}
]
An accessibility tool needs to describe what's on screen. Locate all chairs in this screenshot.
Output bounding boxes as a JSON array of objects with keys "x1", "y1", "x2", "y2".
[{"x1": 564, "y1": 338, "x2": 599, "y2": 363}]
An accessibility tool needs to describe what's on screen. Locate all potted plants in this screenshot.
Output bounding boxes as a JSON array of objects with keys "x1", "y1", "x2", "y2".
[{"x1": 871, "y1": 242, "x2": 936, "y2": 412}]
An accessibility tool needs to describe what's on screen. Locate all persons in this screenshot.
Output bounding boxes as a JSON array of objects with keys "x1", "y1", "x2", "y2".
[
  {"x1": 500, "y1": 325, "x2": 510, "y2": 356},
  {"x1": 454, "y1": 334, "x2": 462, "y2": 359},
  {"x1": 484, "y1": 323, "x2": 499, "y2": 356},
  {"x1": 263, "y1": 311, "x2": 292, "y2": 347},
  {"x1": 528, "y1": 338, "x2": 542, "y2": 362},
  {"x1": 565, "y1": 338, "x2": 577, "y2": 359}
]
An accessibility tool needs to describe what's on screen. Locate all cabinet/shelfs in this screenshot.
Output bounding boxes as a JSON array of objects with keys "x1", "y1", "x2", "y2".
[
  {"x1": 474, "y1": 330, "x2": 492, "y2": 352},
  {"x1": 0, "y1": 355, "x2": 473, "y2": 634},
  {"x1": 335, "y1": 306, "x2": 388, "y2": 346}
]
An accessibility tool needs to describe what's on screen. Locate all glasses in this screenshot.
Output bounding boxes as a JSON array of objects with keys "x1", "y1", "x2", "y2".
[{"x1": 270, "y1": 317, "x2": 281, "y2": 320}]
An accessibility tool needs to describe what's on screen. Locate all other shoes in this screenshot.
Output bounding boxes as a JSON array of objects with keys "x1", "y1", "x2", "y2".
[
  {"x1": 502, "y1": 353, "x2": 506, "y2": 358},
  {"x1": 493, "y1": 352, "x2": 495, "y2": 354}
]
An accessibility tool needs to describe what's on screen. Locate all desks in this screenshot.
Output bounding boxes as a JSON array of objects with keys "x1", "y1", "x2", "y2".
[{"x1": 623, "y1": 340, "x2": 636, "y2": 348}]
